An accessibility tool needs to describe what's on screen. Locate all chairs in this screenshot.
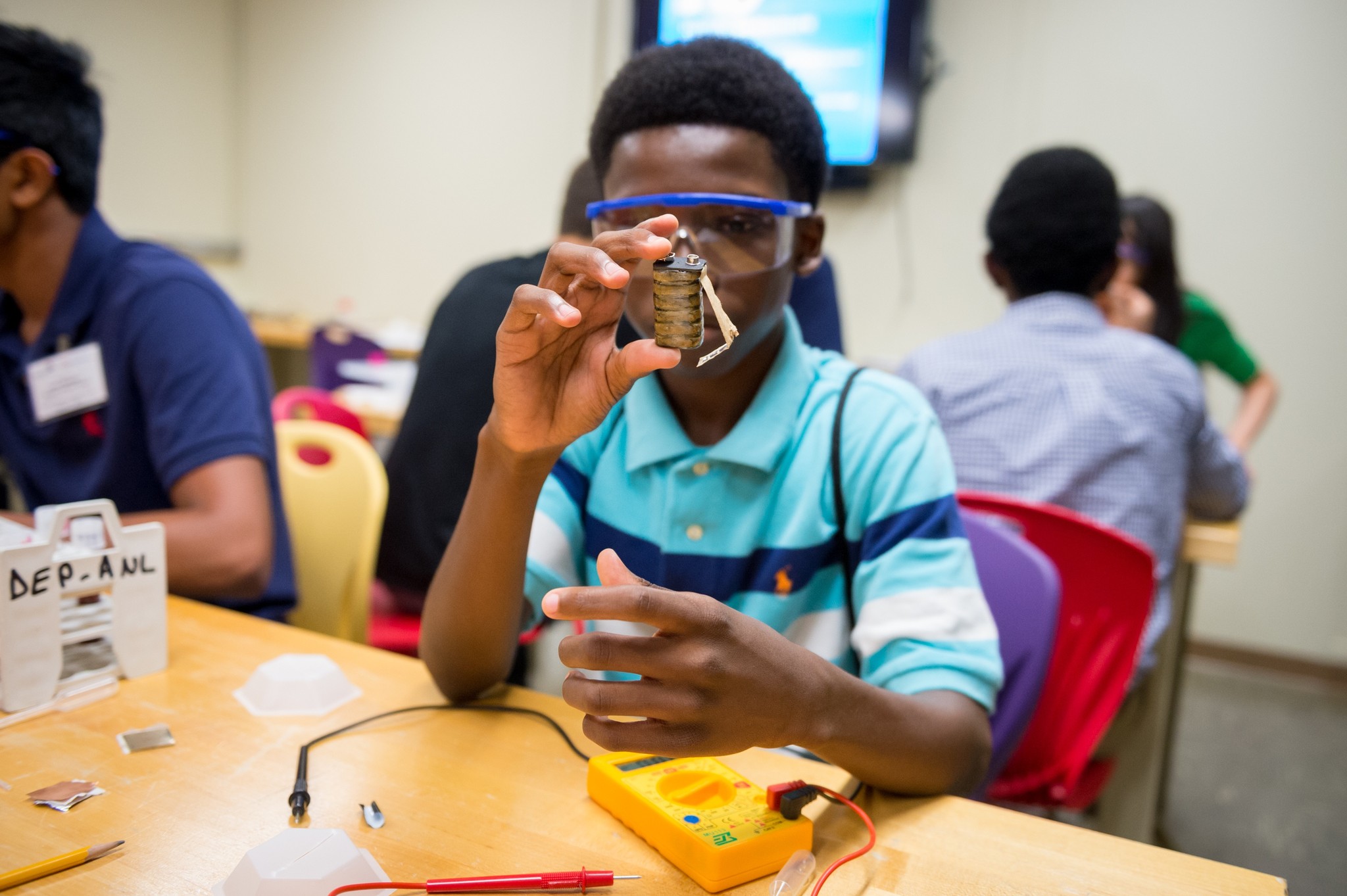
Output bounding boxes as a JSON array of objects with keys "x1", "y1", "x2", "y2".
[
  {"x1": 269, "y1": 387, "x2": 549, "y2": 655},
  {"x1": 957, "y1": 488, "x2": 1160, "y2": 815}
]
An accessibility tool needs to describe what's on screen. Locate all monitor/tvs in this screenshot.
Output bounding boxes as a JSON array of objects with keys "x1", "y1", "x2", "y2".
[{"x1": 635, "y1": 0, "x2": 926, "y2": 187}]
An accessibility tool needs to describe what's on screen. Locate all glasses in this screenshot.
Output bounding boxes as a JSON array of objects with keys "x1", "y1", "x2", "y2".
[{"x1": 584, "y1": 192, "x2": 811, "y2": 282}]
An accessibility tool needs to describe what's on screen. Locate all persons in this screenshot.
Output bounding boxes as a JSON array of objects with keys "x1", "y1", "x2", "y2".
[
  {"x1": 371, "y1": 155, "x2": 604, "y2": 599},
  {"x1": 418, "y1": 37, "x2": 1003, "y2": 799},
  {"x1": 891, "y1": 146, "x2": 1247, "y2": 813},
  {"x1": 1117, "y1": 193, "x2": 1280, "y2": 455},
  {"x1": 0, "y1": 21, "x2": 298, "y2": 625}
]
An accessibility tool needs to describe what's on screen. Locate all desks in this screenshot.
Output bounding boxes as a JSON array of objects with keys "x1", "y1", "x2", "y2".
[{"x1": 3, "y1": 593, "x2": 1290, "y2": 895}]
